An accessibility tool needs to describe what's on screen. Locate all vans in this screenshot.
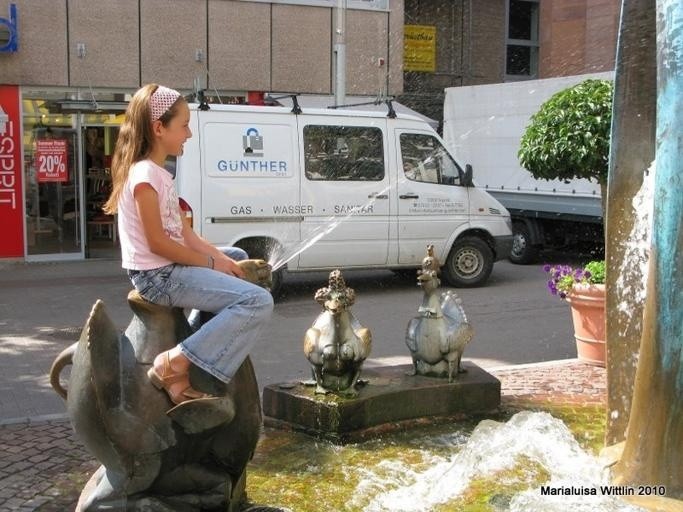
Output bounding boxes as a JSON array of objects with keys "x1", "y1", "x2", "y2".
[{"x1": 161, "y1": 88, "x2": 518, "y2": 305}]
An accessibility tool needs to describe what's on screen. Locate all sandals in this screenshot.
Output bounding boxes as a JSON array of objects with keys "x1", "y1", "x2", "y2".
[{"x1": 147, "y1": 351, "x2": 212, "y2": 406}]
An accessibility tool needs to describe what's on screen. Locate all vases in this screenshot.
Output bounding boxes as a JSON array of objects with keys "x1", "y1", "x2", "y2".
[{"x1": 555, "y1": 283, "x2": 606, "y2": 369}]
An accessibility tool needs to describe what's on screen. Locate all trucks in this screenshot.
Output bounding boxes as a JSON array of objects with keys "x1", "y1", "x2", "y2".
[{"x1": 438, "y1": 71, "x2": 617, "y2": 265}]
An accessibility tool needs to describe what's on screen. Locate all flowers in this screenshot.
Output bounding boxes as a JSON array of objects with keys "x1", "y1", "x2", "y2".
[{"x1": 540, "y1": 258, "x2": 606, "y2": 302}]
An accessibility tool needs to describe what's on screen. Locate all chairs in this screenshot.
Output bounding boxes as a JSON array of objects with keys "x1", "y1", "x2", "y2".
[{"x1": 25, "y1": 165, "x2": 114, "y2": 245}]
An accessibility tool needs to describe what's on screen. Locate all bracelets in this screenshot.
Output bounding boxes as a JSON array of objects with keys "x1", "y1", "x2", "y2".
[{"x1": 208, "y1": 256, "x2": 215, "y2": 270}]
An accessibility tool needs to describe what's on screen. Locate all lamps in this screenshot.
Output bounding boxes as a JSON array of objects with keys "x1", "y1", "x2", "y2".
[{"x1": 77, "y1": 43, "x2": 98, "y2": 109}]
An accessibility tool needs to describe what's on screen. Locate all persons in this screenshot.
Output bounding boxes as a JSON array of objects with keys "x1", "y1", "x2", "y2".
[{"x1": 100, "y1": 84, "x2": 273, "y2": 407}]
